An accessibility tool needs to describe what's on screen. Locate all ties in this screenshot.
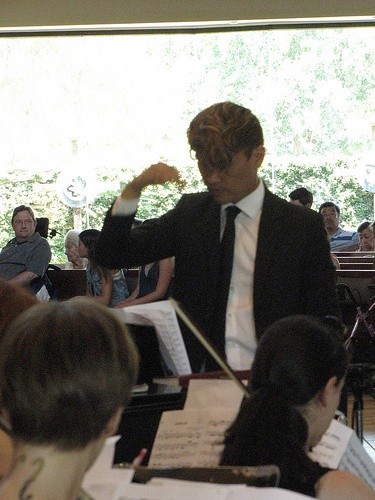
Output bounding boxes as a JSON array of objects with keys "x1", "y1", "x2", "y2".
[{"x1": 205, "y1": 206, "x2": 242, "y2": 372}]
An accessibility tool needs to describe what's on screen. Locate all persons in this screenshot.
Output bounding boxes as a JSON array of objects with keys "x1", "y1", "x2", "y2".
[{"x1": 0, "y1": 101, "x2": 375, "y2": 500}]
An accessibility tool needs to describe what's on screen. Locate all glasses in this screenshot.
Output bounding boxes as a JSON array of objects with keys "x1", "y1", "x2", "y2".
[{"x1": 15, "y1": 220, "x2": 34, "y2": 224}]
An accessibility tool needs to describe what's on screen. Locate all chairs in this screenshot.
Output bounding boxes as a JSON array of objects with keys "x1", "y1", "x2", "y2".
[{"x1": 35, "y1": 218, "x2": 62, "y2": 300}]
